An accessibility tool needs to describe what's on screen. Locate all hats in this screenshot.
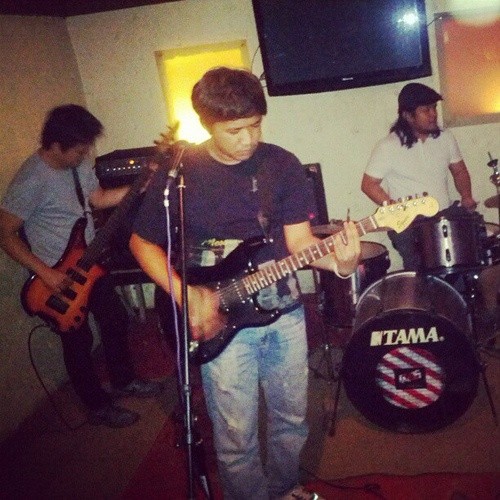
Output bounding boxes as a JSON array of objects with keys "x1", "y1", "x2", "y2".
[{"x1": 398, "y1": 83, "x2": 443, "y2": 107}]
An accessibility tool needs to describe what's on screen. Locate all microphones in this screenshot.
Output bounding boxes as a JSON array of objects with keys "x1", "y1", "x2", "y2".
[{"x1": 165, "y1": 140, "x2": 188, "y2": 187}]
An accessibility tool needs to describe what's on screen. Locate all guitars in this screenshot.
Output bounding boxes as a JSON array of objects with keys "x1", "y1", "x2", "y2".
[
  {"x1": 20, "y1": 120, "x2": 179, "y2": 339},
  {"x1": 153, "y1": 192, "x2": 440, "y2": 367}
]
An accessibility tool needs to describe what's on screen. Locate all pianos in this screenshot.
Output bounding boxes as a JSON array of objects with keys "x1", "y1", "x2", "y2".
[{"x1": 19, "y1": 162, "x2": 341, "y2": 384}]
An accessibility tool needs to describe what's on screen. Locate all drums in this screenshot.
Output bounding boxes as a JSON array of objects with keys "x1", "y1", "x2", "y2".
[
  {"x1": 318, "y1": 240, "x2": 390, "y2": 330},
  {"x1": 340, "y1": 270, "x2": 482, "y2": 437},
  {"x1": 402, "y1": 211, "x2": 492, "y2": 274}
]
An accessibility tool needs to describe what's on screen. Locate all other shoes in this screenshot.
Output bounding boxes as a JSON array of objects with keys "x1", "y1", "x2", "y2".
[
  {"x1": 112, "y1": 377, "x2": 162, "y2": 397},
  {"x1": 88, "y1": 403, "x2": 139, "y2": 425},
  {"x1": 282, "y1": 485, "x2": 326, "y2": 500}
]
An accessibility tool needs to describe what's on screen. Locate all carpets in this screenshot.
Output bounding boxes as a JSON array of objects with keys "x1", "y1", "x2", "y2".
[
  {"x1": 299, "y1": 323, "x2": 500, "y2": 481},
  {"x1": 0, "y1": 374, "x2": 195, "y2": 500}
]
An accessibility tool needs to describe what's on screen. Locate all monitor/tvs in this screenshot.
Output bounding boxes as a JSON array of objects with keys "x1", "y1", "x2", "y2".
[{"x1": 252, "y1": 0, "x2": 432, "y2": 97}]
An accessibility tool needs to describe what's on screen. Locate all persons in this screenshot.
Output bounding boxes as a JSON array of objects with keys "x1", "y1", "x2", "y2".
[
  {"x1": 360, "y1": 83, "x2": 478, "y2": 292},
  {"x1": 0, "y1": 103, "x2": 162, "y2": 430},
  {"x1": 126, "y1": 67, "x2": 361, "y2": 500}
]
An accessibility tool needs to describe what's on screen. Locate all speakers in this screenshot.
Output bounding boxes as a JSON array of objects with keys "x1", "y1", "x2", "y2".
[{"x1": 95, "y1": 144, "x2": 155, "y2": 270}]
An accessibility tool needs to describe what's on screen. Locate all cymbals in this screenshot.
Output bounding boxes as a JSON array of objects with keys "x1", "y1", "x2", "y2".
[{"x1": 484, "y1": 193, "x2": 500, "y2": 210}]
